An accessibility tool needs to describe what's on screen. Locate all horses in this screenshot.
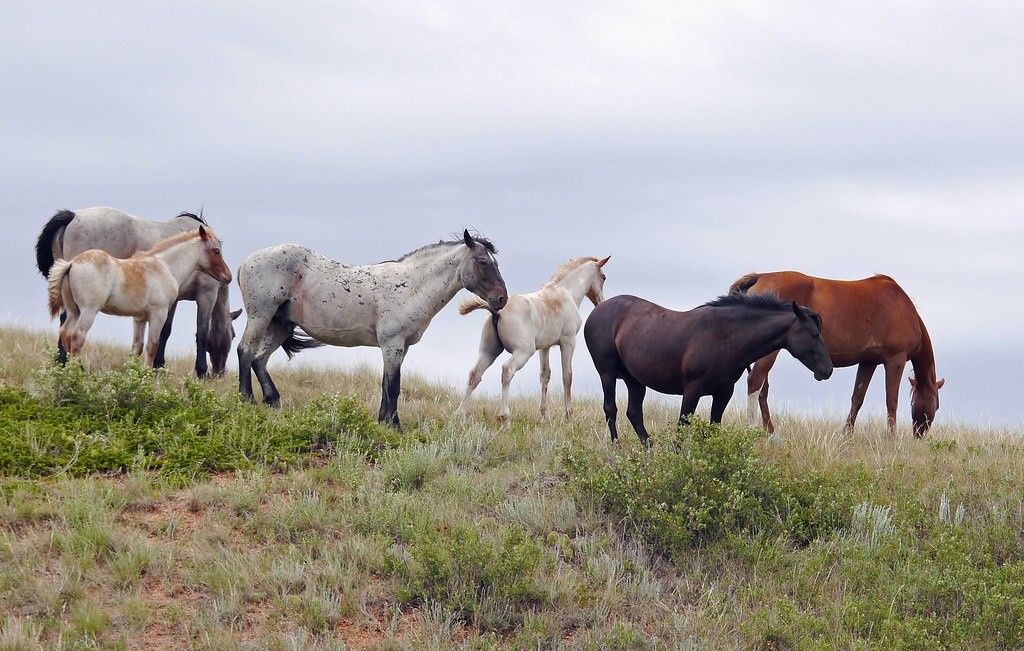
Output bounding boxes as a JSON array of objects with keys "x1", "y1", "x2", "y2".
[
  {"x1": 584, "y1": 291, "x2": 833, "y2": 447},
  {"x1": 36, "y1": 206, "x2": 243, "y2": 381},
  {"x1": 237, "y1": 229, "x2": 509, "y2": 434},
  {"x1": 727, "y1": 271, "x2": 945, "y2": 440},
  {"x1": 458, "y1": 255, "x2": 610, "y2": 429}
]
